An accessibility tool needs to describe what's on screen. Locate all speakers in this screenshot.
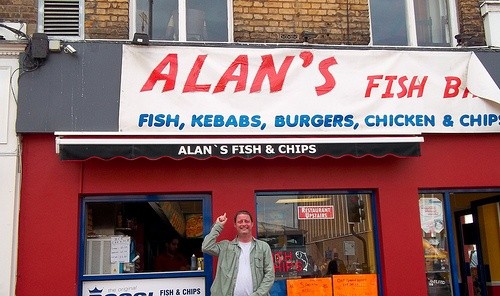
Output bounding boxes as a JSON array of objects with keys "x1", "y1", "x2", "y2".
[{"x1": 31, "y1": 32, "x2": 49, "y2": 58}]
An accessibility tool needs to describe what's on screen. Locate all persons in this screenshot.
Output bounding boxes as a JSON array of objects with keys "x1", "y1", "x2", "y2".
[
  {"x1": 328, "y1": 253, "x2": 347, "y2": 275},
  {"x1": 202, "y1": 211, "x2": 275, "y2": 296},
  {"x1": 301, "y1": 255, "x2": 316, "y2": 278},
  {"x1": 167, "y1": 9, "x2": 207, "y2": 41},
  {"x1": 155, "y1": 232, "x2": 188, "y2": 272}
]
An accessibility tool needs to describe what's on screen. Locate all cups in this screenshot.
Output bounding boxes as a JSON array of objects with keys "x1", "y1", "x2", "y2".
[{"x1": 123, "y1": 263, "x2": 136, "y2": 273}]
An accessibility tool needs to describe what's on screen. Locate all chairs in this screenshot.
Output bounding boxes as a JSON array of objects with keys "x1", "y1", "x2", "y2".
[
  {"x1": 441, "y1": 15, "x2": 451, "y2": 46},
  {"x1": 407, "y1": 16, "x2": 434, "y2": 46}
]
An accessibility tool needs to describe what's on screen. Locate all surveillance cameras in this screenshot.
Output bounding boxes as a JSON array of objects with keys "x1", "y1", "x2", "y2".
[{"x1": 66, "y1": 45, "x2": 77, "y2": 54}]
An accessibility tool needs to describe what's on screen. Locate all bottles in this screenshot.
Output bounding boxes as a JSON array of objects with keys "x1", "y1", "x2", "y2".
[
  {"x1": 191, "y1": 254, "x2": 196, "y2": 270},
  {"x1": 197, "y1": 258, "x2": 204, "y2": 270}
]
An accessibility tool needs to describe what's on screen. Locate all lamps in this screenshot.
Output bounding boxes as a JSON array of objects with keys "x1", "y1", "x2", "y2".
[
  {"x1": 130, "y1": 31, "x2": 152, "y2": 45},
  {"x1": 456, "y1": 32, "x2": 477, "y2": 47},
  {"x1": 298, "y1": 30, "x2": 319, "y2": 45}
]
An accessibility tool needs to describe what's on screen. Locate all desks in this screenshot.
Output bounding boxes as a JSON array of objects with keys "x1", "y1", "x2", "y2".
[{"x1": 172, "y1": 8, "x2": 207, "y2": 43}]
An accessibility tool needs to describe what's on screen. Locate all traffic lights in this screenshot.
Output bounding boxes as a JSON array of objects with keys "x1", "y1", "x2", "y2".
[{"x1": 346, "y1": 194, "x2": 361, "y2": 223}]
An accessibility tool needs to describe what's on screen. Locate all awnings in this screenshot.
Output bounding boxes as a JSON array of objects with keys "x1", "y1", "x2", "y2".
[{"x1": 55, "y1": 134, "x2": 424, "y2": 159}]
